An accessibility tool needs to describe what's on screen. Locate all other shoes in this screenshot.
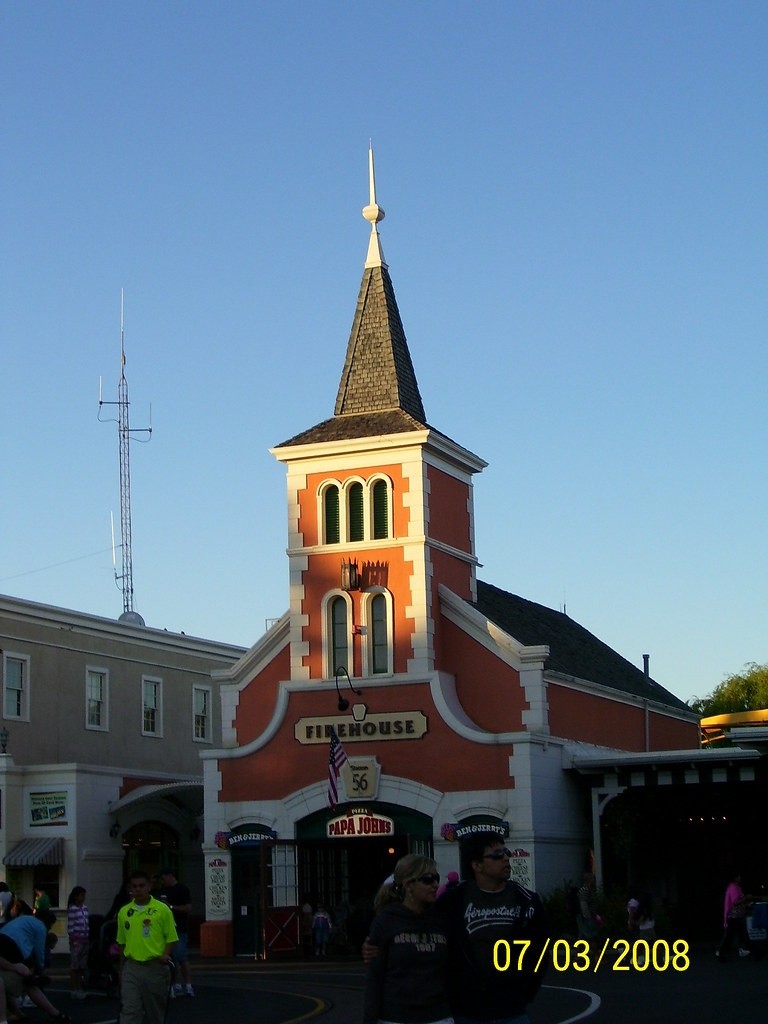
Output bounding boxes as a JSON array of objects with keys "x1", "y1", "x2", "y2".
[
  {"x1": 174, "y1": 987, "x2": 184, "y2": 996},
  {"x1": 322, "y1": 951, "x2": 326, "y2": 956},
  {"x1": 316, "y1": 952, "x2": 319, "y2": 955},
  {"x1": 15, "y1": 994, "x2": 37, "y2": 1007},
  {"x1": 72, "y1": 990, "x2": 90, "y2": 999},
  {"x1": 186, "y1": 984, "x2": 194, "y2": 995},
  {"x1": 739, "y1": 948, "x2": 751, "y2": 957},
  {"x1": 715, "y1": 950, "x2": 723, "y2": 957},
  {"x1": 57, "y1": 1011, "x2": 73, "y2": 1024}
]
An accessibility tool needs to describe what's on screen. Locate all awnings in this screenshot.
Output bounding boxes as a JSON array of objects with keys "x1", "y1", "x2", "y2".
[{"x1": 3, "y1": 837, "x2": 66, "y2": 865}]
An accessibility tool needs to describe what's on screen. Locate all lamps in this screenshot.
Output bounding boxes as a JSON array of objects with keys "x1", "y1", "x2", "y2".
[
  {"x1": 109, "y1": 819, "x2": 121, "y2": 838},
  {"x1": 341, "y1": 557, "x2": 358, "y2": 591},
  {"x1": 336, "y1": 666, "x2": 361, "y2": 711}
]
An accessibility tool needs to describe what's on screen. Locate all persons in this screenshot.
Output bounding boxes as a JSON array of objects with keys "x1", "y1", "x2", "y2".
[
  {"x1": 312, "y1": 904, "x2": 331, "y2": 960},
  {"x1": 67, "y1": 864, "x2": 196, "y2": 1024},
  {"x1": 0, "y1": 881, "x2": 72, "y2": 1024},
  {"x1": 577, "y1": 873, "x2": 594, "y2": 921},
  {"x1": 361, "y1": 827, "x2": 548, "y2": 1024},
  {"x1": 714, "y1": 873, "x2": 751, "y2": 957},
  {"x1": 627, "y1": 891, "x2": 657, "y2": 937}
]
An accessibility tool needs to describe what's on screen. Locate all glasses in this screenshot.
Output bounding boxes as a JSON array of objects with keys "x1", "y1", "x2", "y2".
[
  {"x1": 317, "y1": 906, "x2": 324, "y2": 908},
  {"x1": 481, "y1": 848, "x2": 511, "y2": 860},
  {"x1": 411, "y1": 872, "x2": 440, "y2": 886}
]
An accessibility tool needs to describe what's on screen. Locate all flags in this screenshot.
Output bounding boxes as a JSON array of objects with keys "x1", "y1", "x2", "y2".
[{"x1": 327, "y1": 729, "x2": 348, "y2": 810}]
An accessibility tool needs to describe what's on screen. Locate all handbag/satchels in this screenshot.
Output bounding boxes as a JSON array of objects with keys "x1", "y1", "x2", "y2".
[{"x1": 6, "y1": 891, "x2": 21, "y2": 918}]
